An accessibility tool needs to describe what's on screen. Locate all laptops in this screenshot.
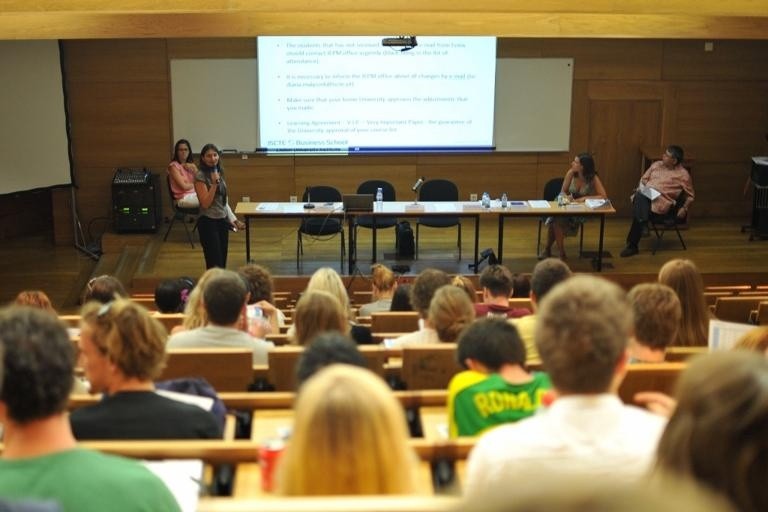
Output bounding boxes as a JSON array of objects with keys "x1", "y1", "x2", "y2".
[{"x1": 343, "y1": 194, "x2": 373, "y2": 212}]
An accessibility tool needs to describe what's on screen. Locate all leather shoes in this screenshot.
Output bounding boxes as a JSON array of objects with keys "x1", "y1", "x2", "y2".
[{"x1": 620, "y1": 244, "x2": 638, "y2": 257}]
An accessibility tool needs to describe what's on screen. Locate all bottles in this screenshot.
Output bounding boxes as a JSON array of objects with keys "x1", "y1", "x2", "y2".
[
  {"x1": 557, "y1": 191, "x2": 564, "y2": 206},
  {"x1": 376, "y1": 188, "x2": 383, "y2": 209},
  {"x1": 501, "y1": 194, "x2": 512, "y2": 211},
  {"x1": 481, "y1": 191, "x2": 491, "y2": 208}
]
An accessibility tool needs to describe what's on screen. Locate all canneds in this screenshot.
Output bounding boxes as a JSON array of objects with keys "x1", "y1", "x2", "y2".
[{"x1": 260, "y1": 438, "x2": 286, "y2": 492}]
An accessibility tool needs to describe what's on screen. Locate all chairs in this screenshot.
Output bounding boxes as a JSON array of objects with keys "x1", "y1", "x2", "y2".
[
  {"x1": 295, "y1": 185, "x2": 346, "y2": 271},
  {"x1": 536, "y1": 178, "x2": 586, "y2": 257},
  {"x1": 635, "y1": 170, "x2": 712, "y2": 256},
  {"x1": 164, "y1": 172, "x2": 200, "y2": 250},
  {"x1": 354, "y1": 180, "x2": 400, "y2": 266},
  {"x1": 412, "y1": 178, "x2": 461, "y2": 262},
  {"x1": 0, "y1": 286, "x2": 768, "y2": 509}
]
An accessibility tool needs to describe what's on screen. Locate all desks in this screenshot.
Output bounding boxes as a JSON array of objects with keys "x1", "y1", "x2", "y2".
[
  {"x1": 490, "y1": 196, "x2": 616, "y2": 274},
  {"x1": 231, "y1": 201, "x2": 349, "y2": 274},
  {"x1": 350, "y1": 200, "x2": 489, "y2": 274}
]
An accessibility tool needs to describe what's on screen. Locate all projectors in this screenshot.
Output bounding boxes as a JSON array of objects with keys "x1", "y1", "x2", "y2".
[{"x1": 382, "y1": 38, "x2": 412, "y2": 46}]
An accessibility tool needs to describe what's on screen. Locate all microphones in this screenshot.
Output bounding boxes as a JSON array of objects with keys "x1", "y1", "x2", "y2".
[
  {"x1": 412, "y1": 176, "x2": 424, "y2": 191},
  {"x1": 212, "y1": 165, "x2": 220, "y2": 183}
]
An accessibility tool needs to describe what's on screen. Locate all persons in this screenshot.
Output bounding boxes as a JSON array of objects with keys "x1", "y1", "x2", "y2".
[
  {"x1": 168, "y1": 139, "x2": 246, "y2": 230},
  {"x1": 537, "y1": 153, "x2": 608, "y2": 261},
  {"x1": 0, "y1": 258, "x2": 768, "y2": 512},
  {"x1": 621, "y1": 145, "x2": 695, "y2": 258},
  {"x1": 194, "y1": 144, "x2": 238, "y2": 271}
]
